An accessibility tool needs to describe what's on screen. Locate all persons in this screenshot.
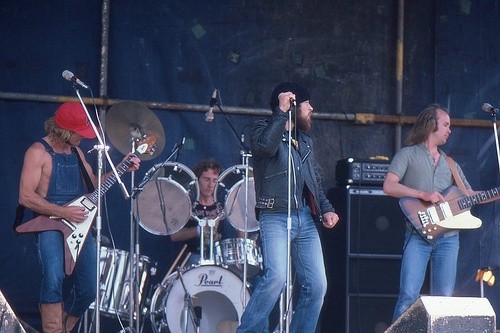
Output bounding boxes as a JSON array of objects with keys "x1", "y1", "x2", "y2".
[
  {"x1": 170, "y1": 158, "x2": 247, "y2": 269},
  {"x1": 236, "y1": 83, "x2": 339, "y2": 333},
  {"x1": 19, "y1": 103, "x2": 140, "y2": 333},
  {"x1": 382, "y1": 105, "x2": 500, "y2": 323}
]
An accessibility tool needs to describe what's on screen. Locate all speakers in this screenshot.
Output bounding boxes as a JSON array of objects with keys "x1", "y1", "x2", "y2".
[
  {"x1": 0, "y1": 290, "x2": 26, "y2": 333},
  {"x1": 326, "y1": 186, "x2": 498, "y2": 333}
]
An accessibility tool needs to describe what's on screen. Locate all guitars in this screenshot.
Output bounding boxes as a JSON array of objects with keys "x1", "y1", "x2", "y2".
[
  {"x1": 15, "y1": 134, "x2": 159, "y2": 276},
  {"x1": 398, "y1": 185, "x2": 500, "y2": 245}
]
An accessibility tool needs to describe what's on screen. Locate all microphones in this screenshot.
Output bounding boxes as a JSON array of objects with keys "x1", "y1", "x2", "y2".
[
  {"x1": 205, "y1": 89, "x2": 217, "y2": 122},
  {"x1": 482, "y1": 103, "x2": 499, "y2": 114},
  {"x1": 62, "y1": 70, "x2": 90, "y2": 90},
  {"x1": 290, "y1": 98, "x2": 296, "y2": 103},
  {"x1": 193, "y1": 306, "x2": 202, "y2": 333},
  {"x1": 240, "y1": 132, "x2": 245, "y2": 165},
  {"x1": 174, "y1": 136, "x2": 186, "y2": 162}
]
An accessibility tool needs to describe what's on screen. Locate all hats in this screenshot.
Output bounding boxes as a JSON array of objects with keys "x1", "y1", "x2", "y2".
[
  {"x1": 54, "y1": 101, "x2": 99, "y2": 139},
  {"x1": 268, "y1": 82, "x2": 310, "y2": 110}
]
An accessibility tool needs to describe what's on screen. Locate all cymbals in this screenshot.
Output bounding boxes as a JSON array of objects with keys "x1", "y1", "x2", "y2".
[{"x1": 105, "y1": 101, "x2": 166, "y2": 162}]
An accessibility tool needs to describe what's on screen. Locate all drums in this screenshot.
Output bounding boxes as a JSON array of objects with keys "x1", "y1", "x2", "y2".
[
  {"x1": 151, "y1": 260, "x2": 251, "y2": 333},
  {"x1": 207, "y1": 238, "x2": 263, "y2": 278},
  {"x1": 212, "y1": 164, "x2": 261, "y2": 232},
  {"x1": 133, "y1": 160, "x2": 200, "y2": 235},
  {"x1": 86, "y1": 246, "x2": 151, "y2": 322}
]
